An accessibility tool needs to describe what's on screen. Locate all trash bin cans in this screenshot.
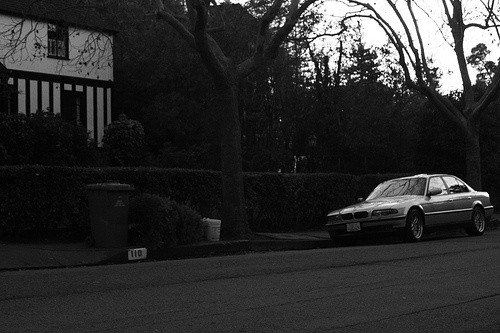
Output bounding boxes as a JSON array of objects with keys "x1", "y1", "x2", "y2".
[{"x1": 87, "y1": 182, "x2": 137, "y2": 249}]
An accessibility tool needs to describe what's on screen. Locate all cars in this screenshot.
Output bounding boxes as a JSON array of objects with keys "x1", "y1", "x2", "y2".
[{"x1": 326, "y1": 172, "x2": 494, "y2": 247}]
